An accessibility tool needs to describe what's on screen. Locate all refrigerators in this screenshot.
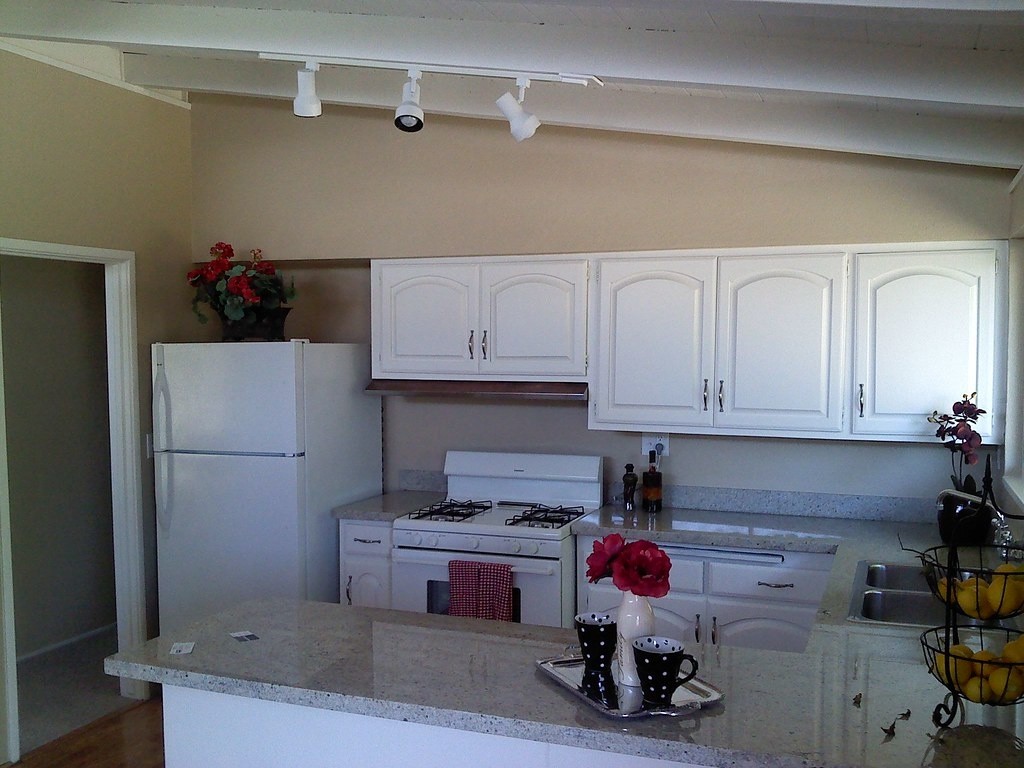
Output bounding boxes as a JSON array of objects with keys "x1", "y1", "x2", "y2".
[{"x1": 148, "y1": 341, "x2": 385, "y2": 634}]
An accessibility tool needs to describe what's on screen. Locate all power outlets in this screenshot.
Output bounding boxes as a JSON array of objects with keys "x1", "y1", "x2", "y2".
[{"x1": 640, "y1": 433, "x2": 669, "y2": 454}]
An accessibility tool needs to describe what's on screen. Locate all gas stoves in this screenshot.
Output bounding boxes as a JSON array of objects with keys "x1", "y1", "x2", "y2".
[{"x1": 391, "y1": 451, "x2": 603, "y2": 559}]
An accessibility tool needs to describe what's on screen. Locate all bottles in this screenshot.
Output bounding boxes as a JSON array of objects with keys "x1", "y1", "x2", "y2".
[
  {"x1": 623, "y1": 463, "x2": 639, "y2": 511},
  {"x1": 642, "y1": 450, "x2": 663, "y2": 513}
]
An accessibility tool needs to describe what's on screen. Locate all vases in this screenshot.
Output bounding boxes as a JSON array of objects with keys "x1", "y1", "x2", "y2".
[
  {"x1": 211, "y1": 305, "x2": 295, "y2": 340},
  {"x1": 616, "y1": 597, "x2": 657, "y2": 688},
  {"x1": 934, "y1": 488, "x2": 999, "y2": 546}
]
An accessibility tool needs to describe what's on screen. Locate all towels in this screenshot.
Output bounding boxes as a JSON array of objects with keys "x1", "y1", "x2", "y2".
[{"x1": 446, "y1": 559, "x2": 516, "y2": 621}]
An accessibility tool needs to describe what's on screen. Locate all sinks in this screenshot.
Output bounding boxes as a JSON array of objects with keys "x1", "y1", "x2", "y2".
[
  {"x1": 860, "y1": 589, "x2": 1004, "y2": 630},
  {"x1": 865, "y1": 563, "x2": 996, "y2": 592}
]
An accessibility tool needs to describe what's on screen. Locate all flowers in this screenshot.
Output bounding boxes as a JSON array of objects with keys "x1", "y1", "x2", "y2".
[
  {"x1": 587, "y1": 529, "x2": 670, "y2": 596},
  {"x1": 188, "y1": 240, "x2": 283, "y2": 302},
  {"x1": 932, "y1": 394, "x2": 983, "y2": 492}
]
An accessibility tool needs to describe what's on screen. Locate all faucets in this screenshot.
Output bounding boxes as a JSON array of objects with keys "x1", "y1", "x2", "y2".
[{"x1": 935, "y1": 488, "x2": 1024, "y2": 563}]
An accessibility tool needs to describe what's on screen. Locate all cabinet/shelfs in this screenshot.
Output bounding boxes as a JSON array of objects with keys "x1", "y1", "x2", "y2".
[
  {"x1": 844, "y1": 633, "x2": 987, "y2": 768},
  {"x1": 850, "y1": 241, "x2": 1006, "y2": 446},
  {"x1": 369, "y1": 254, "x2": 587, "y2": 383},
  {"x1": 588, "y1": 244, "x2": 850, "y2": 439},
  {"x1": 578, "y1": 537, "x2": 834, "y2": 652},
  {"x1": 339, "y1": 517, "x2": 393, "y2": 610}
]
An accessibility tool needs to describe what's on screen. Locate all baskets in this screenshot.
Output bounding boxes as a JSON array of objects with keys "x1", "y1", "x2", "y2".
[
  {"x1": 920, "y1": 539, "x2": 1024, "y2": 622},
  {"x1": 920, "y1": 624, "x2": 1024, "y2": 707}
]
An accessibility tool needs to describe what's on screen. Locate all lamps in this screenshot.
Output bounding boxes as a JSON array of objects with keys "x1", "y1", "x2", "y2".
[
  {"x1": 292, "y1": 62, "x2": 325, "y2": 119},
  {"x1": 495, "y1": 72, "x2": 542, "y2": 140},
  {"x1": 394, "y1": 65, "x2": 426, "y2": 133}
]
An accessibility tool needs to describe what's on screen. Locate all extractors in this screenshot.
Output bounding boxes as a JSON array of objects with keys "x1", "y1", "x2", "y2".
[{"x1": 363, "y1": 379, "x2": 589, "y2": 402}]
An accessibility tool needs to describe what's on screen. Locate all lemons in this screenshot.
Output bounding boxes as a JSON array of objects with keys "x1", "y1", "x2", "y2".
[
  {"x1": 936, "y1": 563, "x2": 1024, "y2": 620},
  {"x1": 936, "y1": 632, "x2": 1024, "y2": 703}
]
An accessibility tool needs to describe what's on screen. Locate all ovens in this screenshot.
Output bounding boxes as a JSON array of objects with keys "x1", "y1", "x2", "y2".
[{"x1": 385, "y1": 548, "x2": 577, "y2": 634}]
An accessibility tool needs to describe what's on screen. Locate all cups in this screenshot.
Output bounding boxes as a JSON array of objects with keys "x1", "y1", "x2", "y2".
[
  {"x1": 632, "y1": 635, "x2": 700, "y2": 706},
  {"x1": 574, "y1": 612, "x2": 618, "y2": 670},
  {"x1": 582, "y1": 669, "x2": 619, "y2": 711}
]
